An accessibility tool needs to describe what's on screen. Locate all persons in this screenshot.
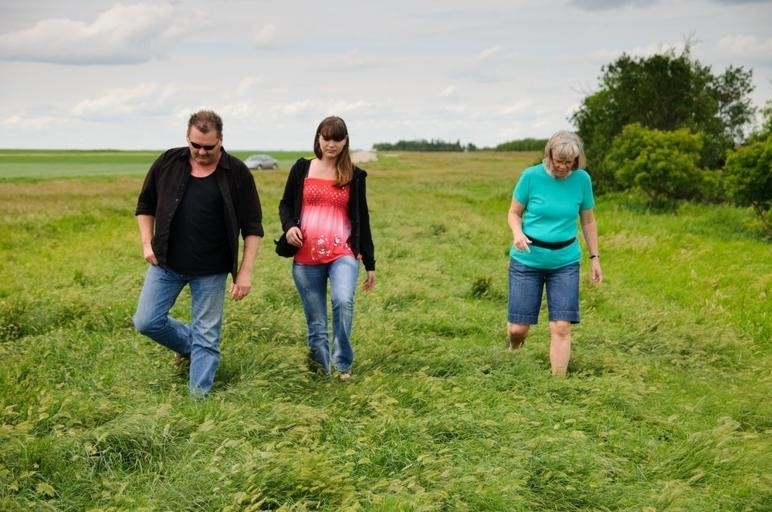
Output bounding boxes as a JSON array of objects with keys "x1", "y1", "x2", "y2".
[
  {"x1": 507, "y1": 130, "x2": 602, "y2": 379},
  {"x1": 134, "y1": 110, "x2": 265, "y2": 396},
  {"x1": 279, "y1": 116, "x2": 378, "y2": 381}
]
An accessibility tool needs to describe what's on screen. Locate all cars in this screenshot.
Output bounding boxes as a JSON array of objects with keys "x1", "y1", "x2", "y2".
[{"x1": 242, "y1": 153, "x2": 279, "y2": 172}]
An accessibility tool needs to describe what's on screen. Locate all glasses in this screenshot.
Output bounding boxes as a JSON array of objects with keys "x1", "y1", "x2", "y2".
[{"x1": 188, "y1": 135, "x2": 221, "y2": 151}]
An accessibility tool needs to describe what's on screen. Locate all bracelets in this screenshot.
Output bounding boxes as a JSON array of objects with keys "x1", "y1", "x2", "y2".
[{"x1": 589, "y1": 255, "x2": 599, "y2": 259}]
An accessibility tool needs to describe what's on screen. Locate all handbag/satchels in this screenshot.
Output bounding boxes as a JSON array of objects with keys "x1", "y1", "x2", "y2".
[{"x1": 273, "y1": 230, "x2": 299, "y2": 258}]
